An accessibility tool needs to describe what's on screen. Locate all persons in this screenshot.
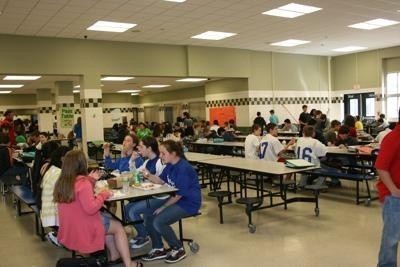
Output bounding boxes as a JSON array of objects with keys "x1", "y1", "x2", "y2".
[
  {"x1": 40, "y1": 145, "x2": 70, "y2": 249},
  {"x1": 0, "y1": 109, "x2": 81, "y2": 207},
  {"x1": 142, "y1": 139, "x2": 202, "y2": 264},
  {"x1": 244, "y1": 105, "x2": 397, "y2": 193},
  {"x1": 107, "y1": 112, "x2": 242, "y2": 158},
  {"x1": 52, "y1": 150, "x2": 143, "y2": 267},
  {"x1": 373, "y1": 107, "x2": 400, "y2": 267},
  {"x1": 125, "y1": 136, "x2": 166, "y2": 249}
]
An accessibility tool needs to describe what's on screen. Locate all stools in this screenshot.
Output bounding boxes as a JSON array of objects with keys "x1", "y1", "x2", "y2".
[{"x1": 178, "y1": 209, "x2": 201, "y2": 253}]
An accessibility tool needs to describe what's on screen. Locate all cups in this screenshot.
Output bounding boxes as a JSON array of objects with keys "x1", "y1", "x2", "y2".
[
  {"x1": 282, "y1": 140, "x2": 286, "y2": 147},
  {"x1": 339, "y1": 145, "x2": 345, "y2": 151}
]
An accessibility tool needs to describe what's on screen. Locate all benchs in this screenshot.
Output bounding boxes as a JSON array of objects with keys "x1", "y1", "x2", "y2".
[{"x1": 0, "y1": 176, "x2": 75, "y2": 267}]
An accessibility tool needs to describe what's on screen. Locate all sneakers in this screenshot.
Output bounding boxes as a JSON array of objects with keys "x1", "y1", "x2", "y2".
[
  {"x1": 44, "y1": 232, "x2": 64, "y2": 248},
  {"x1": 141, "y1": 247, "x2": 167, "y2": 260},
  {"x1": 132, "y1": 238, "x2": 150, "y2": 249},
  {"x1": 129, "y1": 234, "x2": 150, "y2": 244},
  {"x1": 165, "y1": 247, "x2": 186, "y2": 263}
]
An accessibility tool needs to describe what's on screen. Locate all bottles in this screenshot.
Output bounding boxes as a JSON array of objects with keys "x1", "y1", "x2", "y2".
[
  {"x1": 122, "y1": 172, "x2": 129, "y2": 193},
  {"x1": 99, "y1": 167, "x2": 104, "y2": 173},
  {"x1": 137, "y1": 170, "x2": 143, "y2": 183}
]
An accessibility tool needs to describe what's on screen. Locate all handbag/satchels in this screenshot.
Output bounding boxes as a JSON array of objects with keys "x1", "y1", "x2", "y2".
[{"x1": 55, "y1": 254, "x2": 105, "y2": 266}]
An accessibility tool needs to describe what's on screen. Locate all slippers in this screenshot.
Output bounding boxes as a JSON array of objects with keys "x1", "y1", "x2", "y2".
[{"x1": 135, "y1": 262, "x2": 143, "y2": 267}]
